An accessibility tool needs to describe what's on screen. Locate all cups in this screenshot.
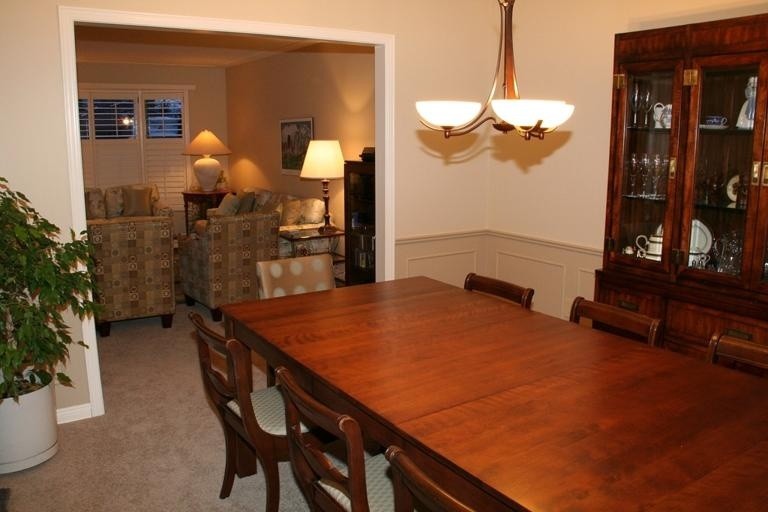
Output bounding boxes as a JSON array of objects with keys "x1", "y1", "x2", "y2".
[{"x1": 705, "y1": 115, "x2": 727, "y2": 124}]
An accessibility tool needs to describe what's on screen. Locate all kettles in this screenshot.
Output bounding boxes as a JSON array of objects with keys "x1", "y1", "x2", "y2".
[{"x1": 735, "y1": 76, "x2": 757, "y2": 128}]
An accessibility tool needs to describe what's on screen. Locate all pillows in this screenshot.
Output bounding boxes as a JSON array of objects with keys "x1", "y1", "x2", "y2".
[
  {"x1": 216, "y1": 192, "x2": 324, "y2": 224},
  {"x1": 122, "y1": 189, "x2": 153, "y2": 217}
]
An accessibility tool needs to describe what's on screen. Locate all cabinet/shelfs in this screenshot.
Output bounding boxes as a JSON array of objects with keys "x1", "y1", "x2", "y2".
[
  {"x1": 593, "y1": 11, "x2": 767, "y2": 362},
  {"x1": 344, "y1": 146, "x2": 375, "y2": 284}
]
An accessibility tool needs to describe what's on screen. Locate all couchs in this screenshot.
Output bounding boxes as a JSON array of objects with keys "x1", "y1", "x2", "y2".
[
  {"x1": 197, "y1": 187, "x2": 339, "y2": 257},
  {"x1": 179, "y1": 212, "x2": 280, "y2": 322},
  {"x1": 83, "y1": 183, "x2": 174, "y2": 216},
  {"x1": 86, "y1": 216, "x2": 176, "y2": 335}
]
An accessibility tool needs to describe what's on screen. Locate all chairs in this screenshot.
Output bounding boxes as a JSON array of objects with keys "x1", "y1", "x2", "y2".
[{"x1": 256, "y1": 253, "x2": 337, "y2": 298}]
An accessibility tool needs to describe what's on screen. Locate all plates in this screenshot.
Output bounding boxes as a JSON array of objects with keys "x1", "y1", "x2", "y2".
[
  {"x1": 724, "y1": 174, "x2": 740, "y2": 200},
  {"x1": 690, "y1": 218, "x2": 712, "y2": 255},
  {"x1": 700, "y1": 124, "x2": 728, "y2": 129}
]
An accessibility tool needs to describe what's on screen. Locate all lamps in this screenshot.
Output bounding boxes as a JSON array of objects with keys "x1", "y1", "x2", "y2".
[
  {"x1": 414, "y1": 1, "x2": 576, "y2": 140},
  {"x1": 299, "y1": 139, "x2": 345, "y2": 235}
]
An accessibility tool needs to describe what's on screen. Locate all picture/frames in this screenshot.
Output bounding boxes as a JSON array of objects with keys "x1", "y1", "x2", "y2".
[
  {"x1": 180, "y1": 127, "x2": 232, "y2": 190},
  {"x1": 279, "y1": 116, "x2": 314, "y2": 177}
]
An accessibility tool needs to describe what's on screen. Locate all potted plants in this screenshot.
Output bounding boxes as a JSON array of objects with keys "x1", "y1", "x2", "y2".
[{"x1": 0, "y1": 175, "x2": 106, "y2": 474}]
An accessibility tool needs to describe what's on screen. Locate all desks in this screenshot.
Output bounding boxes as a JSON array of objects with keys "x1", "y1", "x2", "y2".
[
  {"x1": 180, "y1": 190, "x2": 236, "y2": 236},
  {"x1": 281, "y1": 228, "x2": 344, "y2": 265},
  {"x1": 215, "y1": 275, "x2": 768, "y2": 511}
]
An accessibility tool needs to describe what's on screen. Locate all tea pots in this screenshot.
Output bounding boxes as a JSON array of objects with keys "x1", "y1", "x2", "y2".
[
  {"x1": 688, "y1": 246, "x2": 711, "y2": 271},
  {"x1": 634, "y1": 223, "x2": 664, "y2": 261},
  {"x1": 653, "y1": 103, "x2": 674, "y2": 128}
]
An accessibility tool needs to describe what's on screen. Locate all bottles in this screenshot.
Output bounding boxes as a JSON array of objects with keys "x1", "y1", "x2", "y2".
[
  {"x1": 716, "y1": 233, "x2": 741, "y2": 275},
  {"x1": 627, "y1": 153, "x2": 639, "y2": 191},
  {"x1": 629, "y1": 84, "x2": 640, "y2": 126},
  {"x1": 653, "y1": 156, "x2": 661, "y2": 200},
  {"x1": 642, "y1": 89, "x2": 652, "y2": 125},
  {"x1": 643, "y1": 154, "x2": 650, "y2": 193}
]
